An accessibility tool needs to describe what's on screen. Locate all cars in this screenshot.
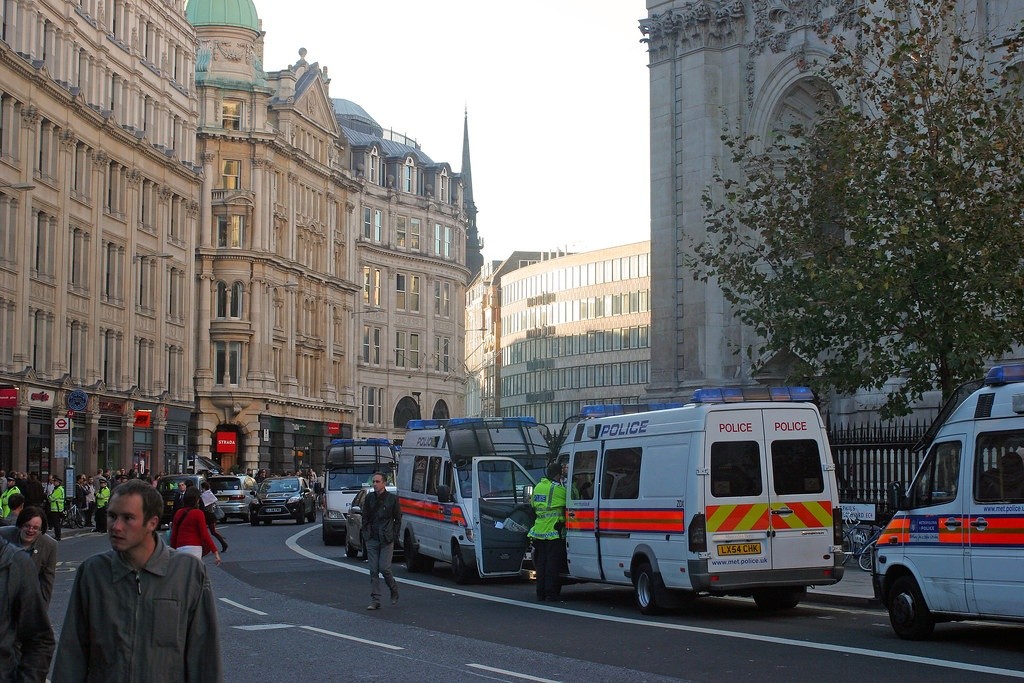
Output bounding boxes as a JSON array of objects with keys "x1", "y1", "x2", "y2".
[{"x1": 246, "y1": 475, "x2": 318, "y2": 527}]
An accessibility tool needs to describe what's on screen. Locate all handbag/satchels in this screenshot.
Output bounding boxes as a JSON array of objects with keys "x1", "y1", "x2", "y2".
[
  {"x1": 213, "y1": 506, "x2": 226, "y2": 520},
  {"x1": 201, "y1": 489, "x2": 217, "y2": 507}
]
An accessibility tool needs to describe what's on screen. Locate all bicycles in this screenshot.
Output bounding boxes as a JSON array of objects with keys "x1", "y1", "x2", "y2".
[
  {"x1": 841, "y1": 515, "x2": 885, "y2": 571},
  {"x1": 61, "y1": 498, "x2": 86, "y2": 529}
]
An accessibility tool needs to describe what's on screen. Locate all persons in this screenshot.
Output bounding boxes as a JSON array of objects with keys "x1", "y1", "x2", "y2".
[
  {"x1": 527, "y1": 464, "x2": 566, "y2": 601},
  {"x1": 0, "y1": 536, "x2": 55, "y2": 683},
  {"x1": 0, "y1": 506, "x2": 58, "y2": 607},
  {"x1": 362, "y1": 473, "x2": 401, "y2": 609},
  {"x1": 170, "y1": 487, "x2": 222, "y2": 567},
  {"x1": 247, "y1": 468, "x2": 270, "y2": 482},
  {"x1": 50, "y1": 480, "x2": 224, "y2": 682},
  {"x1": 200, "y1": 482, "x2": 228, "y2": 552},
  {"x1": 75, "y1": 468, "x2": 165, "y2": 533},
  {"x1": 0, "y1": 470, "x2": 64, "y2": 541},
  {"x1": 170, "y1": 480, "x2": 193, "y2": 513},
  {"x1": 287, "y1": 468, "x2": 325, "y2": 510}
]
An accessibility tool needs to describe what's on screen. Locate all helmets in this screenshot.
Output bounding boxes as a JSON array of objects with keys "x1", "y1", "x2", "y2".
[{"x1": 546, "y1": 462, "x2": 562, "y2": 471}]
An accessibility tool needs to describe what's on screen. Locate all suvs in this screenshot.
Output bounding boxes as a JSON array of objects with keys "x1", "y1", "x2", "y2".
[
  {"x1": 155, "y1": 474, "x2": 206, "y2": 531},
  {"x1": 207, "y1": 473, "x2": 258, "y2": 524}
]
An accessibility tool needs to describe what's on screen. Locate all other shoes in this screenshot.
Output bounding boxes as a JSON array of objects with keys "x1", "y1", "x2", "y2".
[
  {"x1": 84, "y1": 523, "x2": 95, "y2": 527},
  {"x1": 390, "y1": 591, "x2": 399, "y2": 604},
  {"x1": 92, "y1": 529, "x2": 99, "y2": 532},
  {"x1": 54, "y1": 537, "x2": 61, "y2": 541},
  {"x1": 365, "y1": 599, "x2": 381, "y2": 610},
  {"x1": 101, "y1": 531, "x2": 107, "y2": 533},
  {"x1": 221, "y1": 543, "x2": 228, "y2": 553}
]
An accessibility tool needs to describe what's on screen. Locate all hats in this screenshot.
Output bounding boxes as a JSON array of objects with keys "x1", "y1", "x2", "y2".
[
  {"x1": 6, "y1": 476, "x2": 16, "y2": 481},
  {"x1": 99, "y1": 479, "x2": 108, "y2": 483},
  {"x1": 121, "y1": 475, "x2": 129, "y2": 479},
  {"x1": 51, "y1": 477, "x2": 62, "y2": 484}
]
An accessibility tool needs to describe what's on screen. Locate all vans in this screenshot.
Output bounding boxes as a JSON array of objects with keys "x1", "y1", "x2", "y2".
[
  {"x1": 869, "y1": 364, "x2": 1024, "y2": 645},
  {"x1": 393, "y1": 416, "x2": 558, "y2": 585},
  {"x1": 314, "y1": 437, "x2": 403, "y2": 546},
  {"x1": 470, "y1": 385, "x2": 844, "y2": 616}
]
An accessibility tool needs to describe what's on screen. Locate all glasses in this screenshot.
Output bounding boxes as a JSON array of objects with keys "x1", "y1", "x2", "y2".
[{"x1": 22, "y1": 523, "x2": 41, "y2": 532}]
